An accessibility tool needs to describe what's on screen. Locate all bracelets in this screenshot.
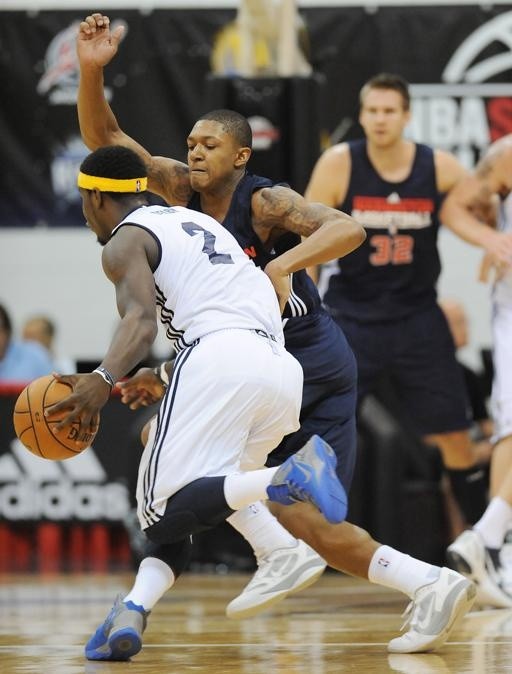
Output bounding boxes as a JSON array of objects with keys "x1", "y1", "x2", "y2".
[
  {"x1": 155, "y1": 363, "x2": 169, "y2": 390},
  {"x1": 93, "y1": 367, "x2": 114, "y2": 392}
]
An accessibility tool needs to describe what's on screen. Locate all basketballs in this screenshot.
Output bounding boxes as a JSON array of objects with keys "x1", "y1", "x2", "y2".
[{"x1": 13, "y1": 374, "x2": 100, "y2": 462}]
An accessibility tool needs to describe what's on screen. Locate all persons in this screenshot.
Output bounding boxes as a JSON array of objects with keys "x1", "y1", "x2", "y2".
[
  {"x1": 1, "y1": 305, "x2": 77, "y2": 382},
  {"x1": 297, "y1": 74, "x2": 511, "y2": 609},
  {"x1": 74, "y1": 13, "x2": 478, "y2": 656},
  {"x1": 42, "y1": 146, "x2": 347, "y2": 660}
]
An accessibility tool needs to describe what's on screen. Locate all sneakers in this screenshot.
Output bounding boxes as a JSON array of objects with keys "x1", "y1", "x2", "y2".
[
  {"x1": 387, "y1": 530, "x2": 512, "y2": 654},
  {"x1": 226, "y1": 538, "x2": 328, "y2": 621},
  {"x1": 266, "y1": 435, "x2": 348, "y2": 526},
  {"x1": 84, "y1": 593, "x2": 152, "y2": 661}
]
